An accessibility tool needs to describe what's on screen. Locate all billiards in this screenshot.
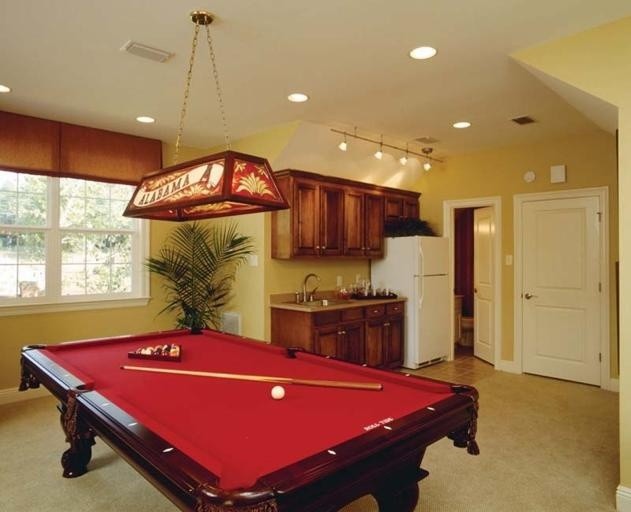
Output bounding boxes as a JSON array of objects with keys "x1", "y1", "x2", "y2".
[
  {"x1": 271, "y1": 386, "x2": 285, "y2": 399},
  {"x1": 135, "y1": 344, "x2": 180, "y2": 356}
]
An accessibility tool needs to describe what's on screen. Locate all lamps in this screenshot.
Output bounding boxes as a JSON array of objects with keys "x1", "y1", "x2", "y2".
[
  {"x1": 122, "y1": 9, "x2": 286, "y2": 220},
  {"x1": 328, "y1": 129, "x2": 444, "y2": 176}
]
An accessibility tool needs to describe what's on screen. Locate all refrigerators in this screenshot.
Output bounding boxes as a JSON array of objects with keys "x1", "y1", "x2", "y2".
[{"x1": 370, "y1": 235, "x2": 451, "y2": 369}]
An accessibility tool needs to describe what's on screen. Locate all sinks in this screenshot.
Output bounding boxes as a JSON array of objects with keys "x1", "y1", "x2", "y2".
[{"x1": 282, "y1": 299, "x2": 355, "y2": 309}]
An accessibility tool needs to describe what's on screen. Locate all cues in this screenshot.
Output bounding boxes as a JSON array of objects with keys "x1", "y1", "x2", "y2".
[{"x1": 120, "y1": 366, "x2": 383, "y2": 390}]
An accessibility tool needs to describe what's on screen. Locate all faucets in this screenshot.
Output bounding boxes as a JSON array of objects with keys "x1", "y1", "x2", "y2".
[{"x1": 302, "y1": 273, "x2": 321, "y2": 302}]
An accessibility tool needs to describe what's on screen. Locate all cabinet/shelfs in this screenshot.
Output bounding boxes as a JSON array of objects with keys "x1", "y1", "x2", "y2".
[
  {"x1": 273, "y1": 309, "x2": 366, "y2": 367},
  {"x1": 272, "y1": 170, "x2": 342, "y2": 260},
  {"x1": 367, "y1": 303, "x2": 404, "y2": 368},
  {"x1": 382, "y1": 187, "x2": 419, "y2": 221},
  {"x1": 342, "y1": 179, "x2": 382, "y2": 259}
]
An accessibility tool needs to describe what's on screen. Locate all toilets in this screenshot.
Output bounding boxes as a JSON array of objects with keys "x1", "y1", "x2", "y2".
[{"x1": 458, "y1": 316, "x2": 473, "y2": 348}]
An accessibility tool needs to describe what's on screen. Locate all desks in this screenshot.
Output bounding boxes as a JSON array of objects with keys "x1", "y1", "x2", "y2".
[{"x1": 13, "y1": 325, "x2": 484, "y2": 512}]
